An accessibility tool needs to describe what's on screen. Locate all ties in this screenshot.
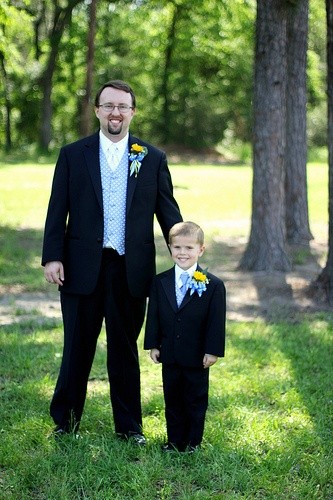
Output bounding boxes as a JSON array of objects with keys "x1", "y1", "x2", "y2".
[
  {"x1": 107, "y1": 143, "x2": 119, "y2": 171},
  {"x1": 180, "y1": 272, "x2": 191, "y2": 295}
]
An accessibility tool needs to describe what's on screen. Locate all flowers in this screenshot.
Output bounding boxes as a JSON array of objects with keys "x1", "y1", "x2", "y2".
[
  {"x1": 127, "y1": 143, "x2": 150, "y2": 178},
  {"x1": 188, "y1": 267, "x2": 212, "y2": 298}
]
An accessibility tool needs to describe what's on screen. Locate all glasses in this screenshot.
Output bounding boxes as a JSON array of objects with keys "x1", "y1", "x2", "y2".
[{"x1": 97, "y1": 103, "x2": 133, "y2": 112}]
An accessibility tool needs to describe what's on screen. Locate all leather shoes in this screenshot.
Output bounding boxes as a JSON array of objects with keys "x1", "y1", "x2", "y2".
[
  {"x1": 53, "y1": 428, "x2": 64, "y2": 438},
  {"x1": 130, "y1": 434, "x2": 146, "y2": 446}
]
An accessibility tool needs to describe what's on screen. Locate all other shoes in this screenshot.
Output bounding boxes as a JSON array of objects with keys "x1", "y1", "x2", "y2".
[{"x1": 161, "y1": 442, "x2": 168, "y2": 452}]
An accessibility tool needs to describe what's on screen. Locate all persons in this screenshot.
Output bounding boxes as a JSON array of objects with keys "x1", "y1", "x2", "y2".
[
  {"x1": 144, "y1": 221, "x2": 227, "y2": 453},
  {"x1": 41, "y1": 81, "x2": 183, "y2": 443}
]
